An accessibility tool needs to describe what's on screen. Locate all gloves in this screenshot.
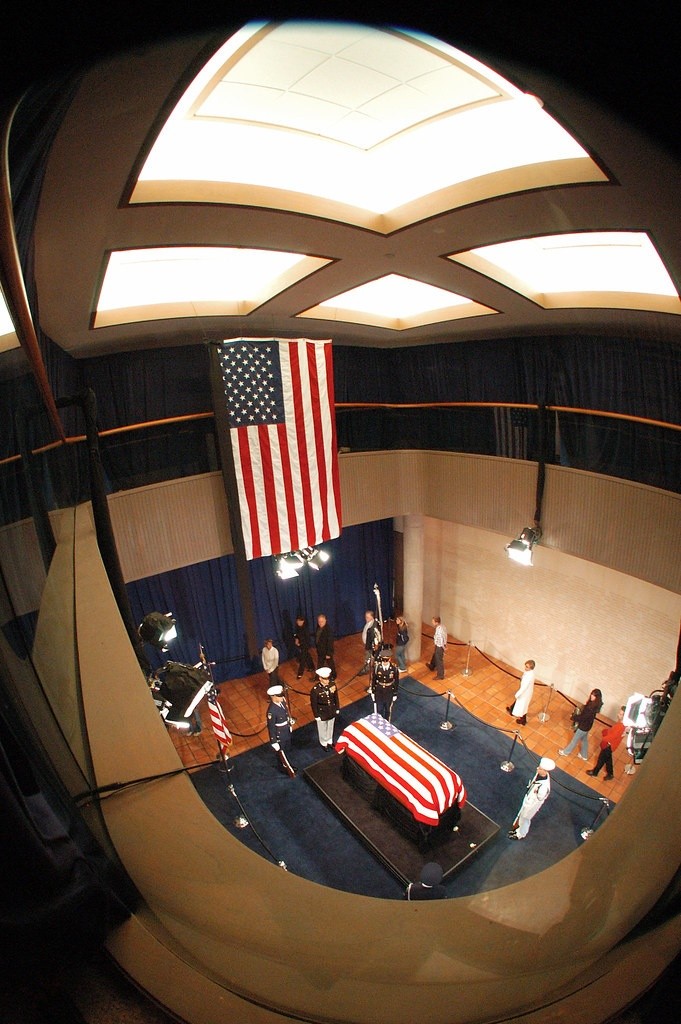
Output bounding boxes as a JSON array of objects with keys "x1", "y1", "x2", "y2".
[
  {"x1": 393, "y1": 696, "x2": 397, "y2": 701},
  {"x1": 315, "y1": 717, "x2": 321, "y2": 722},
  {"x1": 371, "y1": 693, "x2": 375, "y2": 701},
  {"x1": 271, "y1": 743, "x2": 280, "y2": 751},
  {"x1": 336, "y1": 710, "x2": 340, "y2": 714}
]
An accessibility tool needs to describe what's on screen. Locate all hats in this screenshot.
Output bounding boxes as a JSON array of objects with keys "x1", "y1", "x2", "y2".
[
  {"x1": 267, "y1": 686, "x2": 285, "y2": 697},
  {"x1": 316, "y1": 667, "x2": 332, "y2": 679},
  {"x1": 381, "y1": 650, "x2": 393, "y2": 660},
  {"x1": 540, "y1": 758, "x2": 555, "y2": 771}
]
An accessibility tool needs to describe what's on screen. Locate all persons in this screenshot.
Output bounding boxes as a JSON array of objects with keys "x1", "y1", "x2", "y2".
[
  {"x1": 507, "y1": 759, "x2": 556, "y2": 840},
  {"x1": 394, "y1": 618, "x2": 410, "y2": 673},
  {"x1": 587, "y1": 707, "x2": 629, "y2": 781},
  {"x1": 558, "y1": 690, "x2": 603, "y2": 761},
  {"x1": 294, "y1": 614, "x2": 337, "y2": 682},
  {"x1": 372, "y1": 651, "x2": 399, "y2": 722},
  {"x1": 310, "y1": 668, "x2": 342, "y2": 748},
  {"x1": 266, "y1": 686, "x2": 299, "y2": 775},
  {"x1": 360, "y1": 611, "x2": 381, "y2": 675},
  {"x1": 185, "y1": 708, "x2": 201, "y2": 737},
  {"x1": 262, "y1": 640, "x2": 284, "y2": 687},
  {"x1": 426, "y1": 616, "x2": 448, "y2": 681},
  {"x1": 506, "y1": 660, "x2": 535, "y2": 726}
]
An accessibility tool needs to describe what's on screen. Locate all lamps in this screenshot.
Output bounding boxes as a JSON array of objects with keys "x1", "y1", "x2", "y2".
[
  {"x1": 306, "y1": 548, "x2": 330, "y2": 573},
  {"x1": 139, "y1": 613, "x2": 180, "y2": 647},
  {"x1": 148, "y1": 660, "x2": 213, "y2": 725},
  {"x1": 278, "y1": 554, "x2": 304, "y2": 578},
  {"x1": 507, "y1": 525, "x2": 537, "y2": 567},
  {"x1": 623, "y1": 687, "x2": 665, "y2": 756}
]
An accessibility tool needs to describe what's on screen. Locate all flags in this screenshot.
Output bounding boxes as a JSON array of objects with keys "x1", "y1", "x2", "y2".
[
  {"x1": 336, "y1": 714, "x2": 466, "y2": 827},
  {"x1": 212, "y1": 337, "x2": 341, "y2": 562},
  {"x1": 206, "y1": 662, "x2": 233, "y2": 753}
]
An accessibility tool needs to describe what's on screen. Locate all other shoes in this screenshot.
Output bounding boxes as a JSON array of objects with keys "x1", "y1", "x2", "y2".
[
  {"x1": 516, "y1": 719, "x2": 526, "y2": 725},
  {"x1": 604, "y1": 774, "x2": 614, "y2": 780},
  {"x1": 398, "y1": 668, "x2": 407, "y2": 673},
  {"x1": 558, "y1": 750, "x2": 568, "y2": 756},
  {"x1": 586, "y1": 770, "x2": 597, "y2": 776},
  {"x1": 508, "y1": 830, "x2": 516, "y2": 834},
  {"x1": 508, "y1": 834, "x2": 518, "y2": 840},
  {"x1": 329, "y1": 744, "x2": 335, "y2": 749},
  {"x1": 426, "y1": 663, "x2": 433, "y2": 671},
  {"x1": 322, "y1": 746, "x2": 330, "y2": 752},
  {"x1": 578, "y1": 753, "x2": 587, "y2": 760},
  {"x1": 277, "y1": 766, "x2": 298, "y2": 775},
  {"x1": 507, "y1": 707, "x2": 513, "y2": 716},
  {"x1": 432, "y1": 676, "x2": 442, "y2": 680}
]
